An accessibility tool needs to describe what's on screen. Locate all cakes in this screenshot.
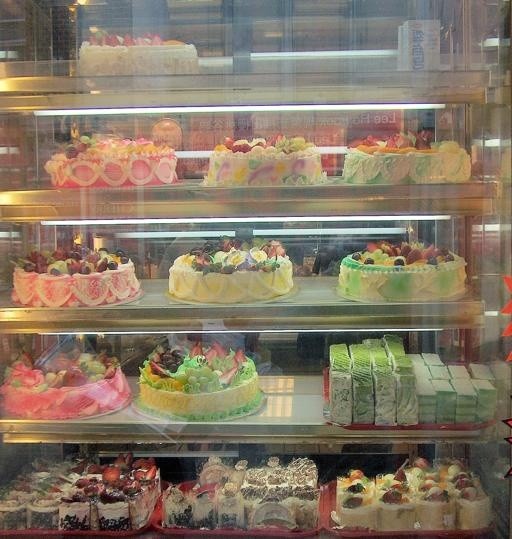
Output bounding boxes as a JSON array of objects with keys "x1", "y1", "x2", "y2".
[
  {"x1": 342, "y1": 131, "x2": 472, "y2": 185},
  {"x1": 336, "y1": 457, "x2": 493, "y2": 531},
  {"x1": 44, "y1": 134, "x2": 178, "y2": 190},
  {"x1": 336, "y1": 239, "x2": 469, "y2": 303},
  {"x1": 136, "y1": 339, "x2": 262, "y2": 422},
  {"x1": 204, "y1": 132, "x2": 322, "y2": 188},
  {"x1": 168, "y1": 235, "x2": 293, "y2": 304},
  {"x1": 78, "y1": 31, "x2": 200, "y2": 77},
  {"x1": 329, "y1": 334, "x2": 498, "y2": 427},
  {"x1": 11, "y1": 243, "x2": 140, "y2": 307},
  {"x1": 1, "y1": 348, "x2": 130, "y2": 420}
]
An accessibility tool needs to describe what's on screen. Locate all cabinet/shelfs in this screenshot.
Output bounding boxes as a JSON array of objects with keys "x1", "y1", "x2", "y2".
[{"x1": 0, "y1": 0, "x2": 512, "y2": 539}]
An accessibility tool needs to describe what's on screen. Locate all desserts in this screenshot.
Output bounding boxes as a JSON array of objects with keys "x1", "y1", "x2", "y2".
[
  {"x1": 162, "y1": 455, "x2": 321, "y2": 531},
  {"x1": 0, "y1": 452, "x2": 162, "y2": 532}
]
